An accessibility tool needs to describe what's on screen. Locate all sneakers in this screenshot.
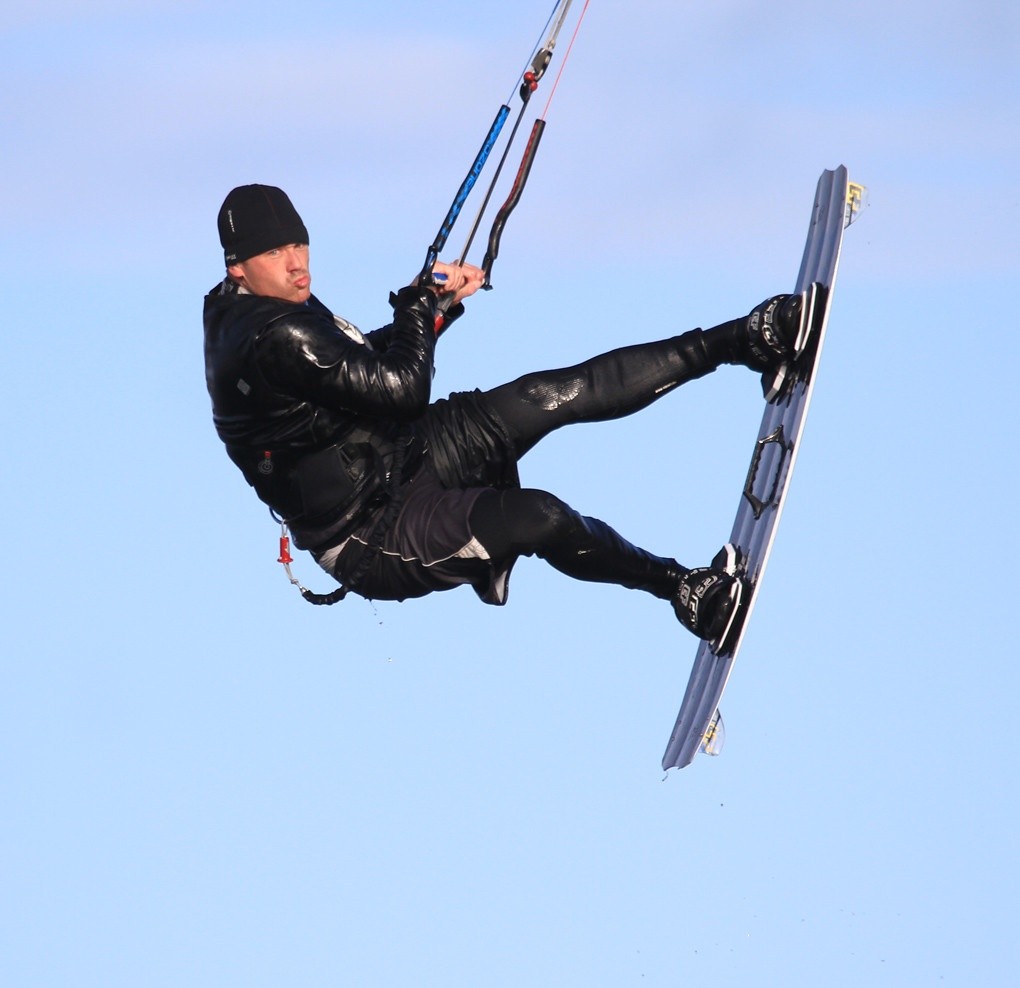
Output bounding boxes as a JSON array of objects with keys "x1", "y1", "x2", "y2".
[
  {"x1": 728, "y1": 295, "x2": 805, "y2": 371},
  {"x1": 660, "y1": 557, "x2": 734, "y2": 640}
]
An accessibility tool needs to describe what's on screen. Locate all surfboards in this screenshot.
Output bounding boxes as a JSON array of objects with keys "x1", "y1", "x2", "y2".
[{"x1": 653, "y1": 162, "x2": 856, "y2": 775}]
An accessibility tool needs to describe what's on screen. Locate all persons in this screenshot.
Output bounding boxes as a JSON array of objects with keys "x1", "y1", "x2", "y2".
[{"x1": 203, "y1": 184, "x2": 809, "y2": 641}]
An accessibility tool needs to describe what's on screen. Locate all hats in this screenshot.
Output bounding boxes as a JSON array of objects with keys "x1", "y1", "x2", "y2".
[{"x1": 217, "y1": 183, "x2": 309, "y2": 267}]
